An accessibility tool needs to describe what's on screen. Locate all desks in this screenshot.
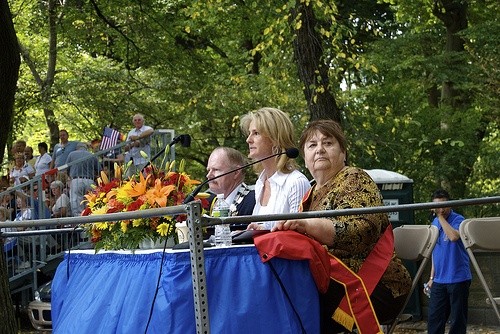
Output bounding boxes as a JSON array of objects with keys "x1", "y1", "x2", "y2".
[{"x1": 51, "y1": 244, "x2": 321, "y2": 334}]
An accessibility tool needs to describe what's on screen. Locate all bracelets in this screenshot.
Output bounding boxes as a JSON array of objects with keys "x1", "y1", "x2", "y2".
[{"x1": 430, "y1": 277, "x2": 433, "y2": 280}]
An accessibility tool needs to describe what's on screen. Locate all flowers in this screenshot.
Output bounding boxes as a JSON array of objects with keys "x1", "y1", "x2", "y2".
[{"x1": 78, "y1": 143, "x2": 212, "y2": 254}]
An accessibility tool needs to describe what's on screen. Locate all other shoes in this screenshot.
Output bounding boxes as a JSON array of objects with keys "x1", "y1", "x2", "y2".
[{"x1": 17, "y1": 261, "x2": 31, "y2": 270}]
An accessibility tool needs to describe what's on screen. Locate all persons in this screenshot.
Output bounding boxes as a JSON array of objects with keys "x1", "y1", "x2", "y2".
[
  {"x1": 0, "y1": 141, "x2": 38, "y2": 232},
  {"x1": 50, "y1": 130, "x2": 91, "y2": 184},
  {"x1": 240, "y1": 107, "x2": 311, "y2": 230},
  {"x1": 35, "y1": 142, "x2": 52, "y2": 175},
  {"x1": 423, "y1": 191, "x2": 473, "y2": 334},
  {"x1": 247, "y1": 119, "x2": 412, "y2": 334},
  {"x1": 201, "y1": 147, "x2": 256, "y2": 227},
  {"x1": 124, "y1": 114, "x2": 154, "y2": 180},
  {"x1": 66, "y1": 142, "x2": 99, "y2": 216},
  {"x1": 15, "y1": 180, "x2": 71, "y2": 273}
]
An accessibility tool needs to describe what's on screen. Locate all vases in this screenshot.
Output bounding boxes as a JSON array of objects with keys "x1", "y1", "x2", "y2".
[{"x1": 137, "y1": 234, "x2": 176, "y2": 250}]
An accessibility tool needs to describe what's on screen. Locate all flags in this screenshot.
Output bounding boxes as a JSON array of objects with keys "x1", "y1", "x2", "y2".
[{"x1": 100, "y1": 127, "x2": 119, "y2": 153}]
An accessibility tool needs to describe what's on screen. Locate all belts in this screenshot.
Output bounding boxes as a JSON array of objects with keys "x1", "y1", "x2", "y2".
[{"x1": 73, "y1": 176, "x2": 94, "y2": 180}]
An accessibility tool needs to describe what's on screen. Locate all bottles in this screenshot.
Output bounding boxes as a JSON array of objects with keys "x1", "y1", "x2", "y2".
[
  {"x1": 424, "y1": 283, "x2": 431, "y2": 298},
  {"x1": 213, "y1": 193, "x2": 232, "y2": 247}
]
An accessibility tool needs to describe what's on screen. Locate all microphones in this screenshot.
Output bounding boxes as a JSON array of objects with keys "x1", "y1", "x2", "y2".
[
  {"x1": 137, "y1": 136, "x2": 192, "y2": 173},
  {"x1": 184, "y1": 148, "x2": 300, "y2": 204}
]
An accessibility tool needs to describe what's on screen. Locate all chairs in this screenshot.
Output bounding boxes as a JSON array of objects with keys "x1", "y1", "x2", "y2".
[
  {"x1": 386, "y1": 225, "x2": 439, "y2": 334},
  {"x1": 459, "y1": 217, "x2": 500, "y2": 320}
]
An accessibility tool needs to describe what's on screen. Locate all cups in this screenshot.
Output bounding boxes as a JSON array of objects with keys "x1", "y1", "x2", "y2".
[{"x1": 176, "y1": 222, "x2": 188, "y2": 244}]
